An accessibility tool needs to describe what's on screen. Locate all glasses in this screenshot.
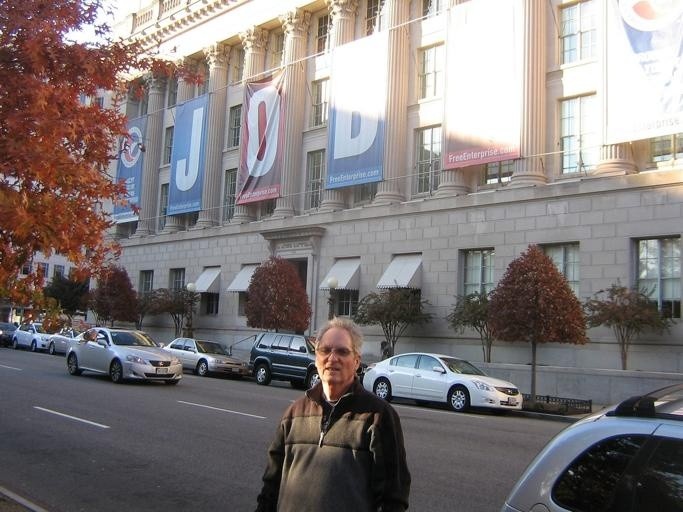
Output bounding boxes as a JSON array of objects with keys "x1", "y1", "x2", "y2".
[{"x1": 317, "y1": 346, "x2": 354, "y2": 356}]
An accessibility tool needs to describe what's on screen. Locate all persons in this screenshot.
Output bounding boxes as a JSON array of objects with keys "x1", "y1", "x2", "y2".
[{"x1": 253, "y1": 317, "x2": 411, "y2": 512}]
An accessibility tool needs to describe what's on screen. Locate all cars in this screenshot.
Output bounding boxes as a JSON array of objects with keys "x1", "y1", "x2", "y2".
[
  {"x1": 0, "y1": 321, "x2": 81, "y2": 354},
  {"x1": 362, "y1": 353, "x2": 523, "y2": 411},
  {"x1": 162, "y1": 337, "x2": 249, "y2": 377},
  {"x1": 501, "y1": 381, "x2": 683, "y2": 512},
  {"x1": 66, "y1": 326, "x2": 183, "y2": 385}
]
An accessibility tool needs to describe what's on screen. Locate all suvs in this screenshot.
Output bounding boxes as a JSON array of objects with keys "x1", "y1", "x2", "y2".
[{"x1": 248, "y1": 332, "x2": 369, "y2": 390}]
